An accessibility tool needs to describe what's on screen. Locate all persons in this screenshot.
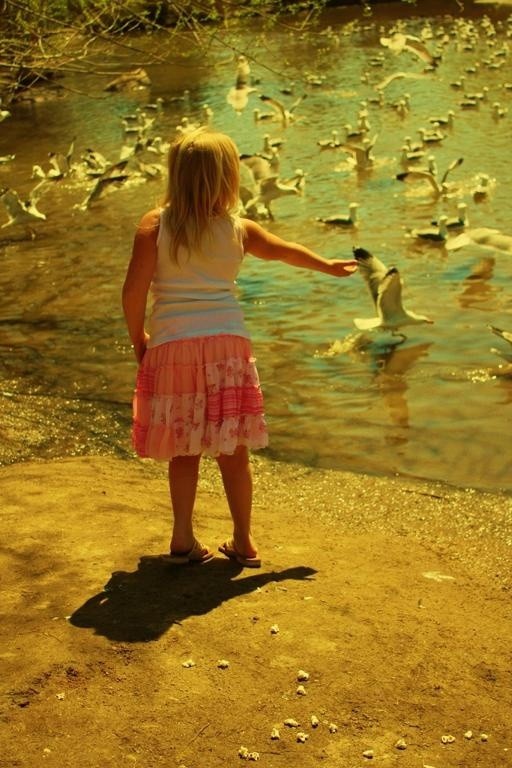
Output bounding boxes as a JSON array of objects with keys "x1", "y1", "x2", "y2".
[{"x1": 121, "y1": 130, "x2": 360, "y2": 568}]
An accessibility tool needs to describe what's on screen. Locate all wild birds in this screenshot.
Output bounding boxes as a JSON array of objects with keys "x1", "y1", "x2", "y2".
[
  {"x1": 315, "y1": 202, "x2": 362, "y2": 228},
  {"x1": 433, "y1": 200, "x2": 469, "y2": 230},
  {"x1": 408, "y1": 214, "x2": 449, "y2": 244},
  {"x1": 317, "y1": 14, "x2": 512, "y2": 200},
  {"x1": 488, "y1": 325, "x2": 512, "y2": 344},
  {"x1": 1, "y1": 55, "x2": 307, "y2": 224},
  {"x1": 352, "y1": 245, "x2": 434, "y2": 332}
]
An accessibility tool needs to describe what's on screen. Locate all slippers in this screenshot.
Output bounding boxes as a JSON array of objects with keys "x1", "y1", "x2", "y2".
[
  {"x1": 218, "y1": 537, "x2": 263, "y2": 568},
  {"x1": 160, "y1": 536, "x2": 216, "y2": 566}
]
